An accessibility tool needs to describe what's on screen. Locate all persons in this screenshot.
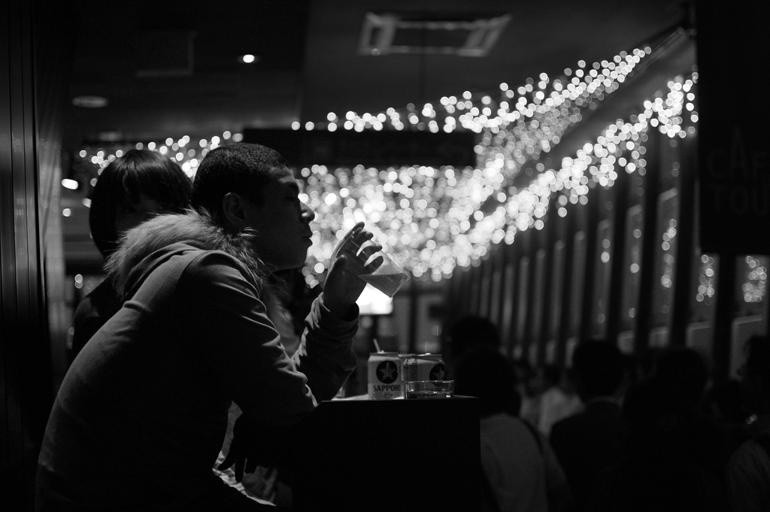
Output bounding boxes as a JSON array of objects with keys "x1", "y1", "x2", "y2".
[
  {"x1": 31, "y1": 142, "x2": 384, "y2": 511},
  {"x1": 443, "y1": 315, "x2": 770, "y2": 512},
  {"x1": 72, "y1": 148, "x2": 303, "y2": 492}
]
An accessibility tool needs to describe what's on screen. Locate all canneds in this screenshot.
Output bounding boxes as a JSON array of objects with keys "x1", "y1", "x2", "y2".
[{"x1": 367, "y1": 352, "x2": 448, "y2": 401}]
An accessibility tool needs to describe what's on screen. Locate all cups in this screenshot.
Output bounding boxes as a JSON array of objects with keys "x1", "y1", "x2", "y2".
[{"x1": 336, "y1": 227, "x2": 409, "y2": 297}]
